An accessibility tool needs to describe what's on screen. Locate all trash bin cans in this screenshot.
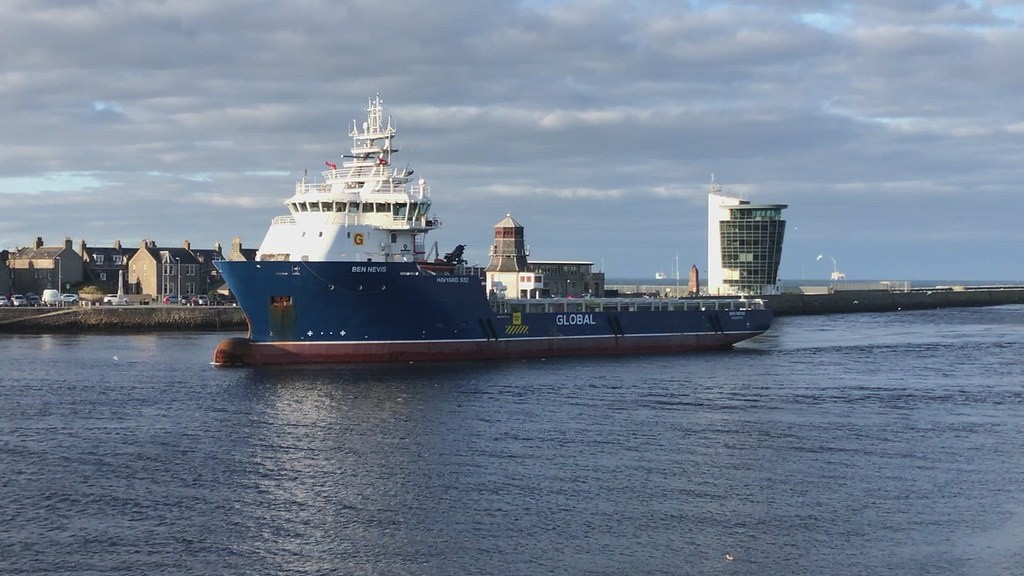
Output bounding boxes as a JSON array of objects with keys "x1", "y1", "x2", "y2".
[
  {"x1": 227, "y1": 300, "x2": 235, "y2": 306},
  {"x1": 140, "y1": 299, "x2": 149, "y2": 305}
]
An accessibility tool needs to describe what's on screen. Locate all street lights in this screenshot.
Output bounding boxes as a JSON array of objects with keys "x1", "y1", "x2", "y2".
[
  {"x1": 56, "y1": 257, "x2": 62, "y2": 297},
  {"x1": 175, "y1": 256, "x2": 181, "y2": 306}
]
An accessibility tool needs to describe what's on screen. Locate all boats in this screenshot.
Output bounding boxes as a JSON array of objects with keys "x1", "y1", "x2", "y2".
[
  {"x1": 415, "y1": 262, "x2": 456, "y2": 275},
  {"x1": 210, "y1": 90, "x2": 788, "y2": 370}
]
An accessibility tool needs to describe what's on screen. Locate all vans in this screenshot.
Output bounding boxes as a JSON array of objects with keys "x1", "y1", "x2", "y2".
[{"x1": 42, "y1": 289, "x2": 60, "y2": 304}]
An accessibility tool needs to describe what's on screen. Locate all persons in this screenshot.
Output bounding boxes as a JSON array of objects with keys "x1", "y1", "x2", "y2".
[
  {"x1": 5, "y1": 290, "x2": 13, "y2": 306},
  {"x1": 165, "y1": 295, "x2": 170, "y2": 305}
]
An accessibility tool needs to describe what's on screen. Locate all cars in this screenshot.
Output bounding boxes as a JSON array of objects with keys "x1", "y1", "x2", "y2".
[
  {"x1": 10, "y1": 295, "x2": 28, "y2": 308},
  {"x1": 164, "y1": 294, "x2": 178, "y2": 305},
  {"x1": 196, "y1": 294, "x2": 209, "y2": 305},
  {"x1": 181, "y1": 294, "x2": 190, "y2": 306},
  {"x1": 26, "y1": 296, "x2": 41, "y2": 307},
  {"x1": 0, "y1": 293, "x2": 10, "y2": 307}
]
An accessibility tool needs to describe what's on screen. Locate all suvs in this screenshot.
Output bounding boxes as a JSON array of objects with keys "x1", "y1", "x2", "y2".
[
  {"x1": 103, "y1": 293, "x2": 128, "y2": 305},
  {"x1": 60, "y1": 294, "x2": 79, "y2": 304}
]
